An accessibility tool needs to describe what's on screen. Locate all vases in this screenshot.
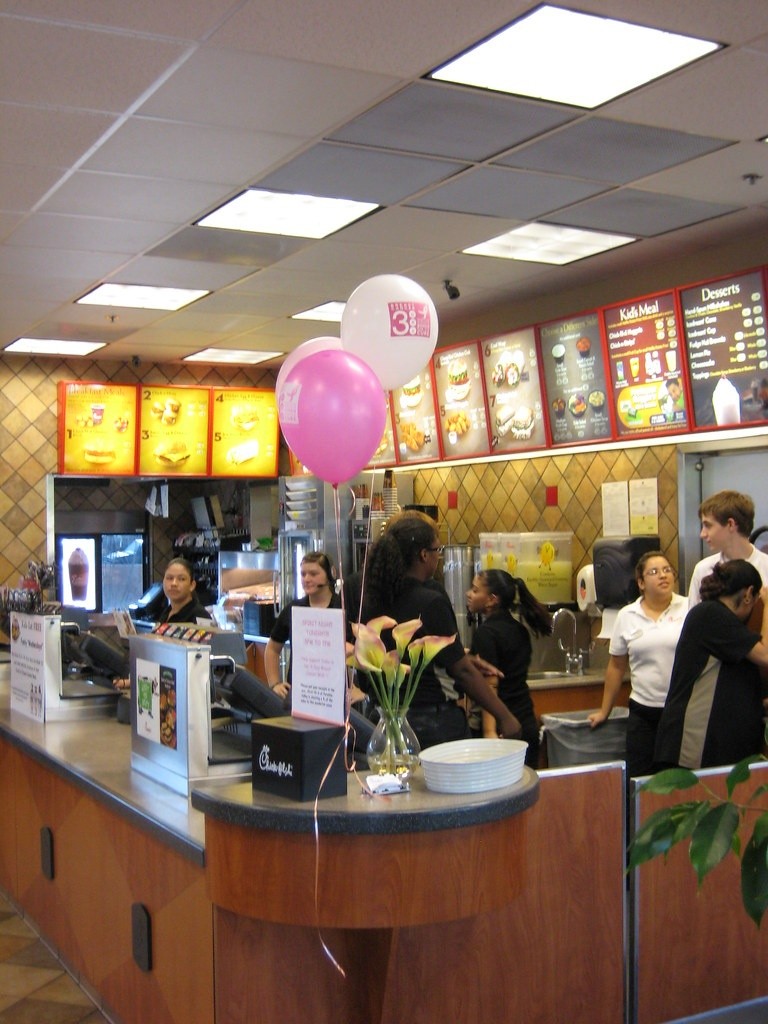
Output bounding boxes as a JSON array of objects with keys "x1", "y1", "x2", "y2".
[{"x1": 365, "y1": 708, "x2": 421, "y2": 775}]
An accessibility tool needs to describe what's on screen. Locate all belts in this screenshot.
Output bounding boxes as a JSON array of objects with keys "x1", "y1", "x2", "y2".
[{"x1": 366, "y1": 696, "x2": 454, "y2": 713}]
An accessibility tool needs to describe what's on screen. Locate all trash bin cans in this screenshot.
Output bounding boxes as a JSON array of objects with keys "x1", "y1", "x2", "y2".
[{"x1": 539, "y1": 706, "x2": 630, "y2": 768}]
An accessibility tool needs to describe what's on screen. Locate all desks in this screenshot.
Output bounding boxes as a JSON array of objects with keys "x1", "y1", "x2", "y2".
[{"x1": 242, "y1": 635, "x2": 291, "y2": 682}]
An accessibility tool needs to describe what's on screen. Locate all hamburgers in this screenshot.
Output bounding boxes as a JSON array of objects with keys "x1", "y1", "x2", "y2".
[
  {"x1": 402, "y1": 374, "x2": 422, "y2": 405},
  {"x1": 447, "y1": 359, "x2": 470, "y2": 392}
]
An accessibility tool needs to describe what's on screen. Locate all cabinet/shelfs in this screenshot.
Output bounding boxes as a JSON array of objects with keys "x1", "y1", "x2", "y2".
[{"x1": 172, "y1": 531, "x2": 250, "y2": 596}]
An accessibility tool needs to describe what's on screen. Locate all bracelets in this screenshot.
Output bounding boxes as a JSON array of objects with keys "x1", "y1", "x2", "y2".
[
  {"x1": 271, "y1": 681, "x2": 280, "y2": 691},
  {"x1": 122, "y1": 678, "x2": 125, "y2": 689}
]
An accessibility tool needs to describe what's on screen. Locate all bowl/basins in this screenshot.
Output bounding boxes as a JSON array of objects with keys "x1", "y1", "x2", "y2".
[
  {"x1": 568, "y1": 394, "x2": 587, "y2": 418},
  {"x1": 416, "y1": 738, "x2": 528, "y2": 794}
]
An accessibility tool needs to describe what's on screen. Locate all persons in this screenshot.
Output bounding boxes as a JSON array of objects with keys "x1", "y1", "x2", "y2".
[
  {"x1": 687, "y1": 492, "x2": 768, "y2": 761},
  {"x1": 665, "y1": 380, "x2": 687, "y2": 421},
  {"x1": 113, "y1": 558, "x2": 212, "y2": 689},
  {"x1": 264, "y1": 510, "x2": 520, "y2": 753},
  {"x1": 587, "y1": 550, "x2": 687, "y2": 787},
  {"x1": 653, "y1": 559, "x2": 768, "y2": 772},
  {"x1": 465, "y1": 569, "x2": 555, "y2": 771}
]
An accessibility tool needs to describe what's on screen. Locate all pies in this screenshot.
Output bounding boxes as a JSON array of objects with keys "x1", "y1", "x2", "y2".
[{"x1": 491, "y1": 349, "x2": 526, "y2": 386}]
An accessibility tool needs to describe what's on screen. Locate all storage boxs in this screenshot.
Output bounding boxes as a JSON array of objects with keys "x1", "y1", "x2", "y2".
[
  {"x1": 477, "y1": 531, "x2": 576, "y2": 603},
  {"x1": 251, "y1": 717, "x2": 347, "y2": 802}
]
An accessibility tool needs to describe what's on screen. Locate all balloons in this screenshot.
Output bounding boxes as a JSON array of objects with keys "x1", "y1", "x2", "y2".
[{"x1": 274, "y1": 271, "x2": 439, "y2": 486}]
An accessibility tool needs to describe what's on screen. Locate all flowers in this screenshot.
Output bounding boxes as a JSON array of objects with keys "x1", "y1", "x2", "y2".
[{"x1": 349, "y1": 616, "x2": 458, "y2": 758}]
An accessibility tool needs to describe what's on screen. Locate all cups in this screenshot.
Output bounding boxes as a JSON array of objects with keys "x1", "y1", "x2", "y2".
[
  {"x1": 589, "y1": 390, "x2": 605, "y2": 413},
  {"x1": 89, "y1": 403, "x2": 105, "y2": 422},
  {"x1": 382, "y1": 470, "x2": 399, "y2": 518},
  {"x1": 666, "y1": 350, "x2": 676, "y2": 373},
  {"x1": 68, "y1": 565, "x2": 89, "y2": 600},
  {"x1": 576, "y1": 337, "x2": 592, "y2": 357},
  {"x1": 552, "y1": 345, "x2": 566, "y2": 364},
  {"x1": 615, "y1": 356, "x2": 624, "y2": 382},
  {"x1": 629, "y1": 357, "x2": 638, "y2": 379},
  {"x1": 552, "y1": 399, "x2": 565, "y2": 419}
]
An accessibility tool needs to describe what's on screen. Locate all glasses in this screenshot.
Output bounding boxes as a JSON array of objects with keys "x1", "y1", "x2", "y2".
[
  {"x1": 428, "y1": 545, "x2": 445, "y2": 553},
  {"x1": 644, "y1": 567, "x2": 672, "y2": 577}
]
